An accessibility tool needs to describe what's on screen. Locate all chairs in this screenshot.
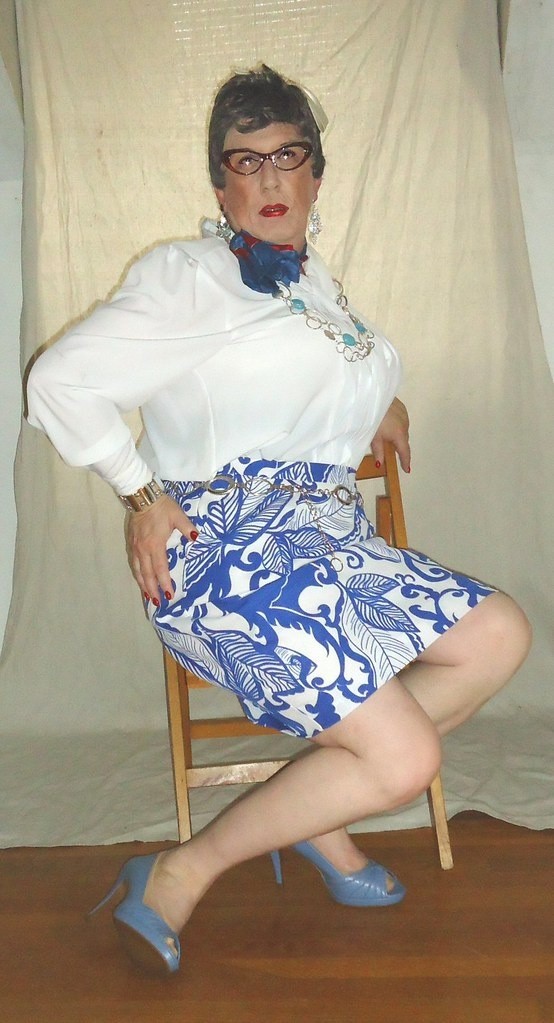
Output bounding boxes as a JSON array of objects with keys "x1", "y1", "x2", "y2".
[{"x1": 163, "y1": 440, "x2": 455, "y2": 872}]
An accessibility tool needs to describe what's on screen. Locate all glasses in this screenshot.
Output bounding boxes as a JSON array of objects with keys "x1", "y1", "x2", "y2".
[{"x1": 219, "y1": 142, "x2": 313, "y2": 176}]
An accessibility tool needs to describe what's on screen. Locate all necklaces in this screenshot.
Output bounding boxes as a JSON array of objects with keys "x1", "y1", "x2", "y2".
[{"x1": 216, "y1": 215, "x2": 375, "y2": 362}]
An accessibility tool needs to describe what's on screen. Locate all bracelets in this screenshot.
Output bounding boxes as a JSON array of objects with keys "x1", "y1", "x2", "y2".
[{"x1": 117, "y1": 471, "x2": 167, "y2": 513}]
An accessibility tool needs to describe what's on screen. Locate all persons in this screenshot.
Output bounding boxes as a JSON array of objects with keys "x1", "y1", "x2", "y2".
[{"x1": 25, "y1": 64, "x2": 532, "y2": 986}]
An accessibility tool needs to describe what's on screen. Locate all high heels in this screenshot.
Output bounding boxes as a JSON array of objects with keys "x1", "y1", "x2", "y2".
[
  {"x1": 87, "y1": 851, "x2": 181, "y2": 976},
  {"x1": 269, "y1": 840, "x2": 405, "y2": 906}
]
